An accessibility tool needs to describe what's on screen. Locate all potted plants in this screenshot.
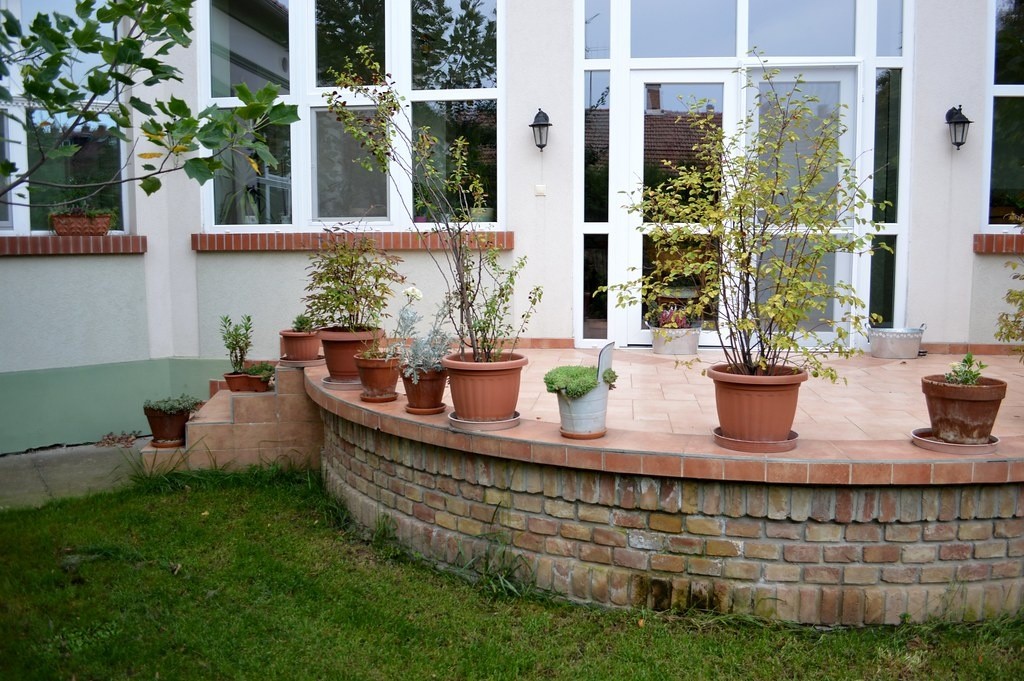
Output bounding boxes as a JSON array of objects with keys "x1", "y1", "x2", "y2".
[
  {"x1": 385, "y1": 291, "x2": 460, "y2": 409},
  {"x1": 321, "y1": 43, "x2": 544, "y2": 430},
  {"x1": 143, "y1": 392, "x2": 202, "y2": 447},
  {"x1": 598, "y1": 44, "x2": 896, "y2": 453},
  {"x1": 217, "y1": 152, "x2": 273, "y2": 224},
  {"x1": 49, "y1": 176, "x2": 117, "y2": 236},
  {"x1": 543, "y1": 340, "x2": 619, "y2": 440},
  {"x1": 300, "y1": 219, "x2": 408, "y2": 389},
  {"x1": 911, "y1": 352, "x2": 1007, "y2": 452},
  {"x1": 220, "y1": 314, "x2": 276, "y2": 391},
  {"x1": 279, "y1": 314, "x2": 327, "y2": 367}
]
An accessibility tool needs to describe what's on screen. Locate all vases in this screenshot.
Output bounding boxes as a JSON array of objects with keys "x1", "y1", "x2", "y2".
[
  {"x1": 354, "y1": 352, "x2": 402, "y2": 403},
  {"x1": 651, "y1": 326, "x2": 699, "y2": 354}
]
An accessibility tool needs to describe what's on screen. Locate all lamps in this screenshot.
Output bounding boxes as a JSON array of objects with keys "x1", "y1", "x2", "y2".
[
  {"x1": 529, "y1": 107, "x2": 552, "y2": 151},
  {"x1": 945, "y1": 104, "x2": 973, "y2": 150}
]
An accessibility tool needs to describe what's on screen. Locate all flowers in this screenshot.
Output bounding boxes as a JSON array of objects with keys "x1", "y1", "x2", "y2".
[
  {"x1": 646, "y1": 301, "x2": 697, "y2": 328},
  {"x1": 351, "y1": 285, "x2": 422, "y2": 359}
]
